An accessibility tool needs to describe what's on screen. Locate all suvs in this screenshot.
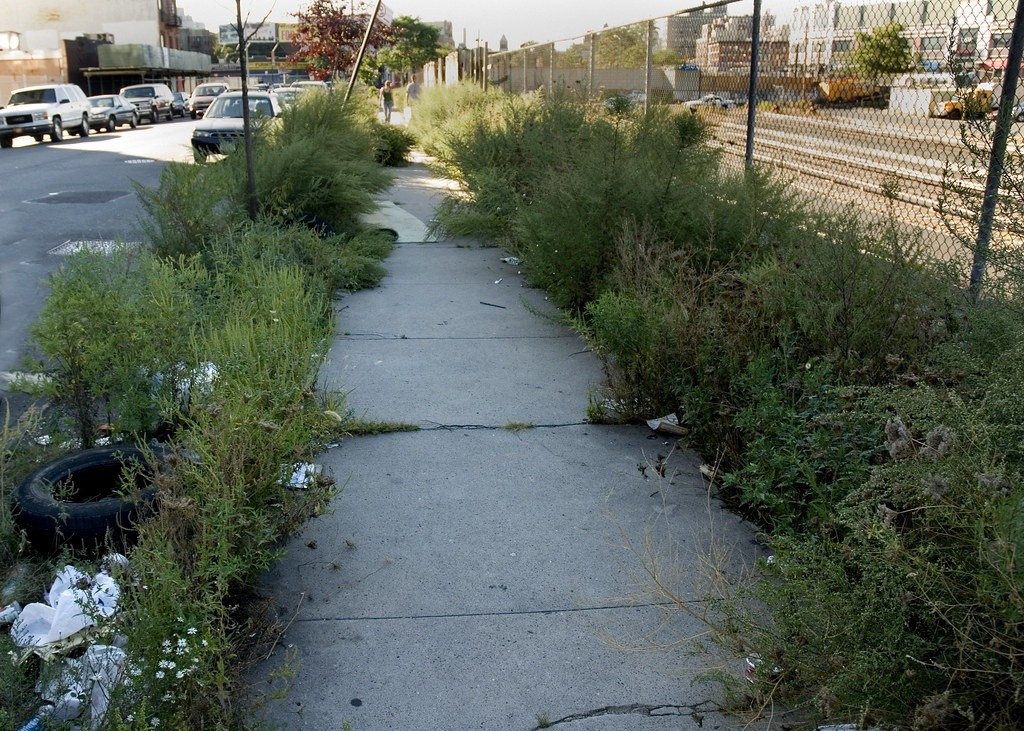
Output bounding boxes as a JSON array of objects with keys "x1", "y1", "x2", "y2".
[
  {"x1": 120, "y1": 83, "x2": 175, "y2": 124},
  {"x1": 0, "y1": 83, "x2": 93, "y2": 148}
]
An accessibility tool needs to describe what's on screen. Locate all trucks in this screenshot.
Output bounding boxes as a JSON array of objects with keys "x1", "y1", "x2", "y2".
[
  {"x1": 935, "y1": 78, "x2": 1024, "y2": 119},
  {"x1": 818, "y1": 79, "x2": 881, "y2": 104}
]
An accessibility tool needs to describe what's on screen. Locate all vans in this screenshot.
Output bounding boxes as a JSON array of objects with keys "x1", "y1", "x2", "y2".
[{"x1": 189, "y1": 83, "x2": 231, "y2": 119}]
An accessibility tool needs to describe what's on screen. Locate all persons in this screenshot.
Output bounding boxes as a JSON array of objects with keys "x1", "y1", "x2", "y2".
[
  {"x1": 406, "y1": 75, "x2": 421, "y2": 106},
  {"x1": 379, "y1": 80, "x2": 394, "y2": 122}
]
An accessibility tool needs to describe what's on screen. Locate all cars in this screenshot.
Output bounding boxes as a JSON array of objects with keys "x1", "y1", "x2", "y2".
[
  {"x1": 251, "y1": 80, "x2": 338, "y2": 113},
  {"x1": 684, "y1": 94, "x2": 736, "y2": 114},
  {"x1": 172, "y1": 92, "x2": 191, "y2": 117},
  {"x1": 191, "y1": 90, "x2": 292, "y2": 163},
  {"x1": 679, "y1": 64, "x2": 698, "y2": 70},
  {"x1": 985, "y1": 109, "x2": 998, "y2": 119},
  {"x1": 86, "y1": 95, "x2": 140, "y2": 132},
  {"x1": 1011, "y1": 102, "x2": 1024, "y2": 121}
]
